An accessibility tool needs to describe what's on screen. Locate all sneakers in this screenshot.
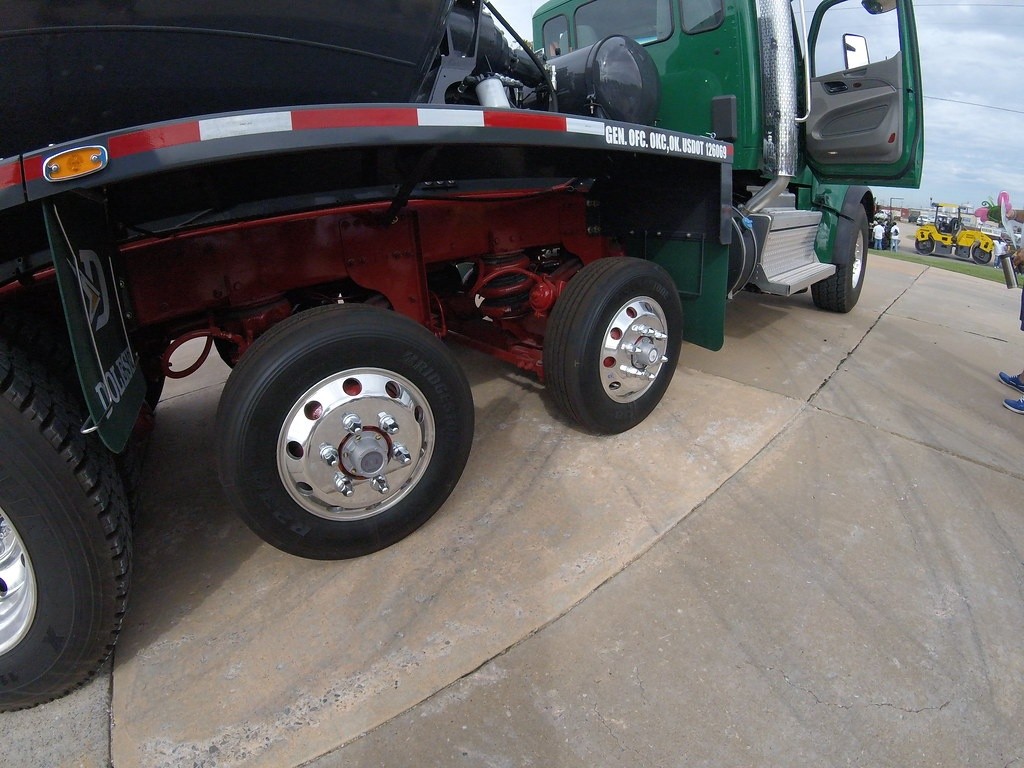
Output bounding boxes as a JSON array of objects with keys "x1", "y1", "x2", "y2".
[
  {"x1": 998, "y1": 370, "x2": 1024, "y2": 394},
  {"x1": 1003, "y1": 397, "x2": 1024, "y2": 414}
]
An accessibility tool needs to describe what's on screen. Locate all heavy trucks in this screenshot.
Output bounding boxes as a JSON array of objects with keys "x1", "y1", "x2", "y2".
[{"x1": 0, "y1": 0, "x2": 925, "y2": 717}]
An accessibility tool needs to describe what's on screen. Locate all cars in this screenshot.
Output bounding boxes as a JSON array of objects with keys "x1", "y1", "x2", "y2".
[{"x1": 916, "y1": 214, "x2": 930, "y2": 227}]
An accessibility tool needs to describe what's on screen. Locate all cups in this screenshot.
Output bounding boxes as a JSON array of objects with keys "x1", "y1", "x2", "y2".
[{"x1": 999, "y1": 253, "x2": 1018, "y2": 289}]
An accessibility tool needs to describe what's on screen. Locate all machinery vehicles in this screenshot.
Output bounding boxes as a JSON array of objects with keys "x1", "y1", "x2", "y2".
[{"x1": 913, "y1": 197, "x2": 994, "y2": 265}]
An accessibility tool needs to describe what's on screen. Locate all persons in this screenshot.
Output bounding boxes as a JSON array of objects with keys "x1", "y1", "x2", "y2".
[
  {"x1": 891, "y1": 222, "x2": 900, "y2": 252},
  {"x1": 998, "y1": 247, "x2": 1024, "y2": 415},
  {"x1": 993, "y1": 237, "x2": 1006, "y2": 269},
  {"x1": 873, "y1": 221, "x2": 885, "y2": 250}
]
